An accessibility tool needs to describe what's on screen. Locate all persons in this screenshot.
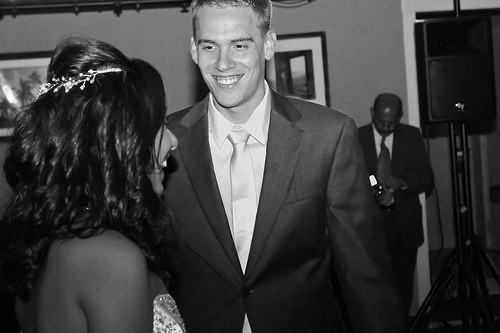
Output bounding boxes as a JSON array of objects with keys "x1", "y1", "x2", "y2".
[
  {"x1": 357, "y1": 93, "x2": 434, "y2": 315},
  {"x1": 159, "y1": 0, "x2": 406, "y2": 333},
  {"x1": 0, "y1": 36, "x2": 186, "y2": 333}
]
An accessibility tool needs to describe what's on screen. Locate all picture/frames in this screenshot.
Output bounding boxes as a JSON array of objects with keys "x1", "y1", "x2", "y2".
[
  {"x1": 263, "y1": 31, "x2": 332, "y2": 108},
  {"x1": 0, "y1": 49, "x2": 58, "y2": 143}
]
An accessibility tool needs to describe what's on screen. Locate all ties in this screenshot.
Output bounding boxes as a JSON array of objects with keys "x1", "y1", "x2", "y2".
[
  {"x1": 226, "y1": 128, "x2": 256, "y2": 273},
  {"x1": 377, "y1": 137, "x2": 392, "y2": 205}
]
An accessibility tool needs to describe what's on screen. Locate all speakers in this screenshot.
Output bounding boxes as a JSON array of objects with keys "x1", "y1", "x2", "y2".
[{"x1": 413, "y1": 16, "x2": 497, "y2": 126}]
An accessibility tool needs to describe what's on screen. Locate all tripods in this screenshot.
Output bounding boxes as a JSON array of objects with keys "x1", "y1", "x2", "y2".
[{"x1": 407, "y1": 121, "x2": 500, "y2": 333}]
